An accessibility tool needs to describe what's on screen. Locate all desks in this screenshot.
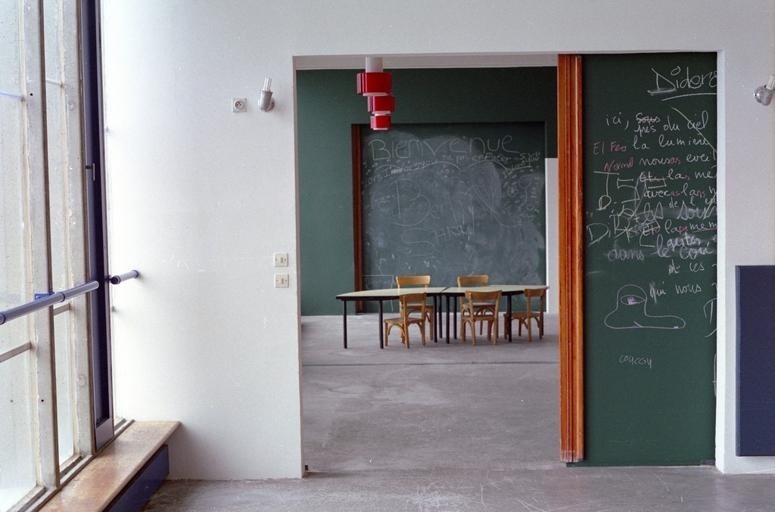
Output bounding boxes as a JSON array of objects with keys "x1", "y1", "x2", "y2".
[{"x1": 335, "y1": 285, "x2": 550, "y2": 349}]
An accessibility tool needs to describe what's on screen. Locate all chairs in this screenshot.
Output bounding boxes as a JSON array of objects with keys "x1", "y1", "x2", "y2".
[{"x1": 383, "y1": 275, "x2": 545, "y2": 350}]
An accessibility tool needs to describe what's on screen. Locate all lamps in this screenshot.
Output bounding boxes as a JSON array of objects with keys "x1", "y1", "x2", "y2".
[
  {"x1": 356, "y1": 56, "x2": 396, "y2": 131},
  {"x1": 755, "y1": 75, "x2": 775, "y2": 106},
  {"x1": 257, "y1": 78, "x2": 275, "y2": 113}
]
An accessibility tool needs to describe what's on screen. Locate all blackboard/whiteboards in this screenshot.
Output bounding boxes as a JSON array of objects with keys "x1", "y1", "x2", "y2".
[
  {"x1": 351, "y1": 122, "x2": 546, "y2": 312},
  {"x1": 558, "y1": 50, "x2": 717, "y2": 467}
]
